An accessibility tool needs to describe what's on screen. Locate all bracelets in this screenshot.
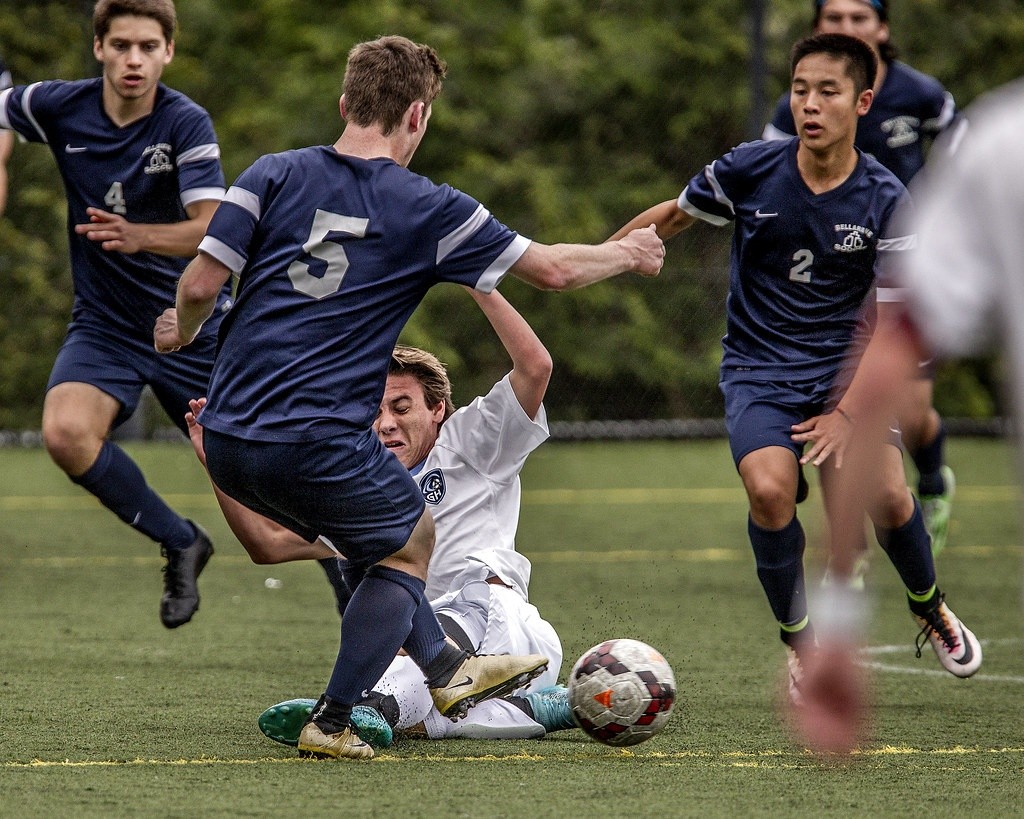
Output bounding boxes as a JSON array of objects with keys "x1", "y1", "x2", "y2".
[{"x1": 836, "y1": 407, "x2": 857, "y2": 426}]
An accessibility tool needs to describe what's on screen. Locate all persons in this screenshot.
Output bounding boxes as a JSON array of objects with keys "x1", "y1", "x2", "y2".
[
  {"x1": 762, "y1": 1, "x2": 969, "y2": 582},
  {"x1": 154, "y1": 37, "x2": 665, "y2": 763},
  {"x1": 609, "y1": 30, "x2": 984, "y2": 709},
  {"x1": 0, "y1": 0, "x2": 349, "y2": 631},
  {"x1": 184, "y1": 285, "x2": 584, "y2": 746}
]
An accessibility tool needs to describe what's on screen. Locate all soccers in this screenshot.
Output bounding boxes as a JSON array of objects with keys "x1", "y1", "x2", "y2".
[{"x1": 568, "y1": 638, "x2": 677, "y2": 747}]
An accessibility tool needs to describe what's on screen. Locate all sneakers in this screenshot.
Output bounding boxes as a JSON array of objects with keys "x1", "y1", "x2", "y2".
[
  {"x1": 820, "y1": 549, "x2": 871, "y2": 591},
  {"x1": 299, "y1": 721, "x2": 376, "y2": 762},
  {"x1": 258, "y1": 695, "x2": 393, "y2": 752},
  {"x1": 428, "y1": 641, "x2": 551, "y2": 723},
  {"x1": 781, "y1": 638, "x2": 823, "y2": 708},
  {"x1": 917, "y1": 466, "x2": 955, "y2": 559},
  {"x1": 907, "y1": 593, "x2": 983, "y2": 678},
  {"x1": 526, "y1": 684, "x2": 577, "y2": 734},
  {"x1": 159, "y1": 516, "x2": 214, "y2": 628}
]
